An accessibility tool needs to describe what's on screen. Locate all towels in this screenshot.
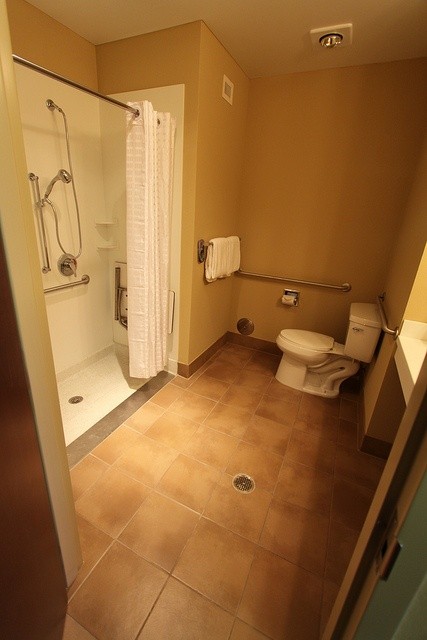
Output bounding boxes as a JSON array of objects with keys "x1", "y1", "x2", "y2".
[
  {"x1": 205, "y1": 238, "x2": 229, "y2": 282},
  {"x1": 228, "y1": 235, "x2": 240, "y2": 276}
]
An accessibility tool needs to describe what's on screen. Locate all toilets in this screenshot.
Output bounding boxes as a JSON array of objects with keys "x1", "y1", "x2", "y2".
[{"x1": 275, "y1": 302, "x2": 383, "y2": 398}]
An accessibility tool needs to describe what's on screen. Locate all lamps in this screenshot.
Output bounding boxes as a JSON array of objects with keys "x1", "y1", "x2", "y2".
[{"x1": 309, "y1": 22, "x2": 353, "y2": 51}]
